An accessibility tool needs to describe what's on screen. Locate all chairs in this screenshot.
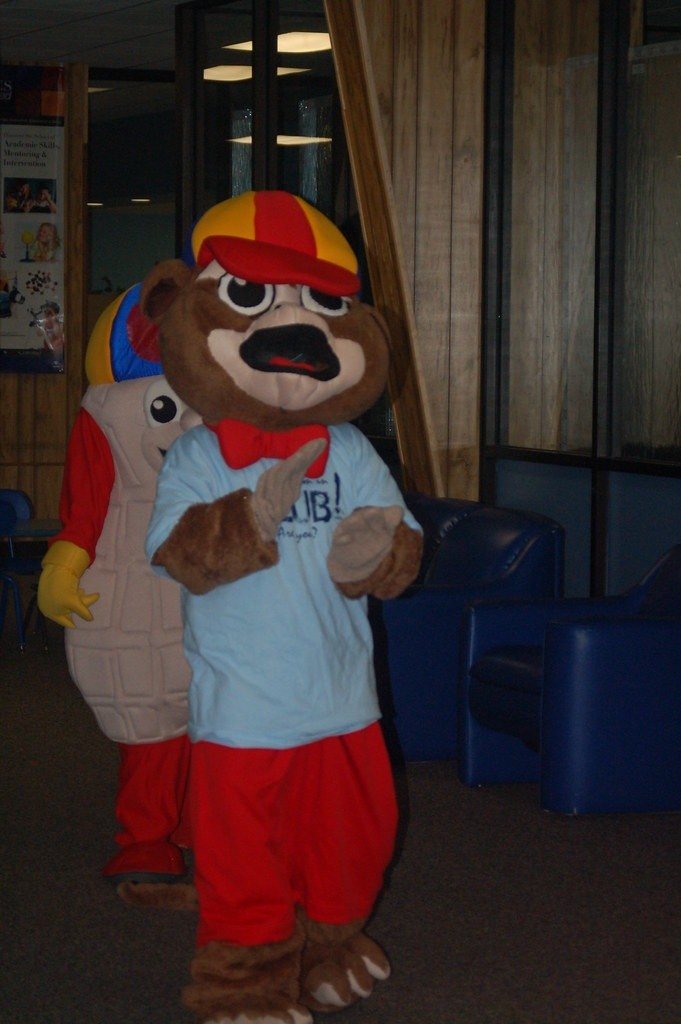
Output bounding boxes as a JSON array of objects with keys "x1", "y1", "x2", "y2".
[{"x1": 0, "y1": 487, "x2": 71, "y2": 655}]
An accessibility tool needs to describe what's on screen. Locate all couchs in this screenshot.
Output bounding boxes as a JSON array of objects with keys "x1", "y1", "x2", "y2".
[
  {"x1": 366, "y1": 488, "x2": 565, "y2": 761},
  {"x1": 456, "y1": 539, "x2": 680, "y2": 817}
]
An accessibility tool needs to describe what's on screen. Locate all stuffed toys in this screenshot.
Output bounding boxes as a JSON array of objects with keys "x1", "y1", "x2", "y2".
[
  {"x1": 140, "y1": 189, "x2": 430, "y2": 1024},
  {"x1": 37, "y1": 246, "x2": 214, "y2": 913}
]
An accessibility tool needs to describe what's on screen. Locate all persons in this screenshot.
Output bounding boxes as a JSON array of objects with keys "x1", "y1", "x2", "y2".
[
  {"x1": 6, "y1": 181, "x2": 58, "y2": 213},
  {"x1": 27, "y1": 223, "x2": 60, "y2": 261},
  {"x1": 36, "y1": 301, "x2": 65, "y2": 348}
]
